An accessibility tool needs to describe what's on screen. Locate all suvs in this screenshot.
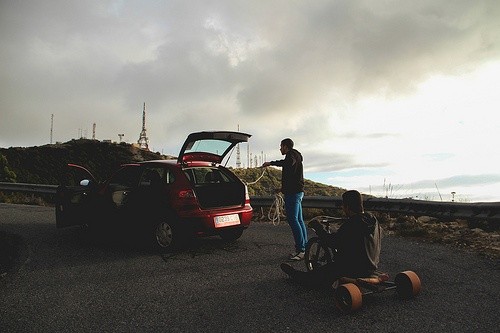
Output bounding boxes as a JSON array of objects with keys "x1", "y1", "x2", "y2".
[{"x1": 56, "y1": 130, "x2": 254, "y2": 251}]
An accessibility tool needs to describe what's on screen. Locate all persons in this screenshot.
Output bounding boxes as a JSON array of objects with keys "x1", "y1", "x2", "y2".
[
  {"x1": 280, "y1": 190, "x2": 383, "y2": 284},
  {"x1": 261, "y1": 138, "x2": 310, "y2": 261}
]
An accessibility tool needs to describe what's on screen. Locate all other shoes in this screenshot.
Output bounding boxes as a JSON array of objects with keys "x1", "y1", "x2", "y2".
[
  {"x1": 279, "y1": 263, "x2": 295, "y2": 276},
  {"x1": 289, "y1": 250, "x2": 306, "y2": 260}
]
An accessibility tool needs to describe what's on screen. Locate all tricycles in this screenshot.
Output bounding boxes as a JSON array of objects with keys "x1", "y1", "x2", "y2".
[{"x1": 304, "y1": 215, "x2": 421, "y2": 314}]
